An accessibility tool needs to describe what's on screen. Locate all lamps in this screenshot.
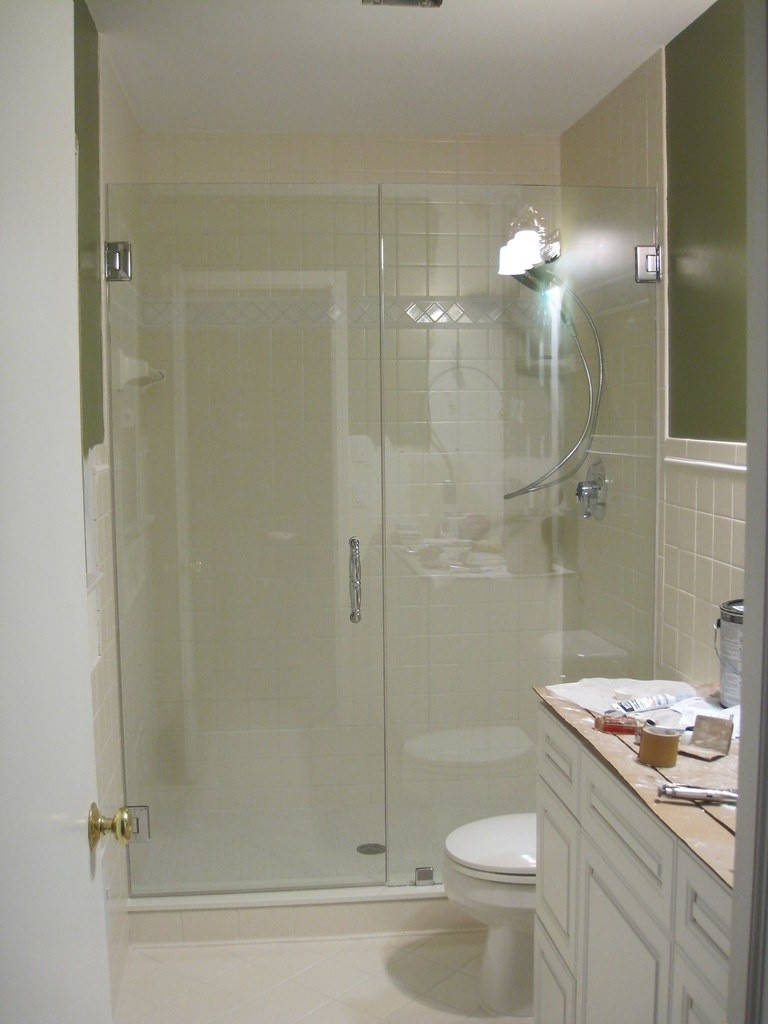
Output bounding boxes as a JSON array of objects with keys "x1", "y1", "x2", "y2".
[{"x1": 496, "y1": 229, "x2": 555, "y2": 294}]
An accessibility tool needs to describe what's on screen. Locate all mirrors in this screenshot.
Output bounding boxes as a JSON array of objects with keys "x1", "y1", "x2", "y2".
[{"x1": 664, "y1": 0, "x2": 747, "y2": 447}]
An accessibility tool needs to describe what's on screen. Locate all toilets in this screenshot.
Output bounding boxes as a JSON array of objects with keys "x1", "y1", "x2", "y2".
[{"x1": 440, "y1": 803, "x2": 538, "y2": 1019}]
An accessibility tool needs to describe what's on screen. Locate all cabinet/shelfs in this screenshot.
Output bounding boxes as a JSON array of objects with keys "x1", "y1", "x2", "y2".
[{"x1": 531, "y1": 700, "x2": 733, "y2": 1024}]
